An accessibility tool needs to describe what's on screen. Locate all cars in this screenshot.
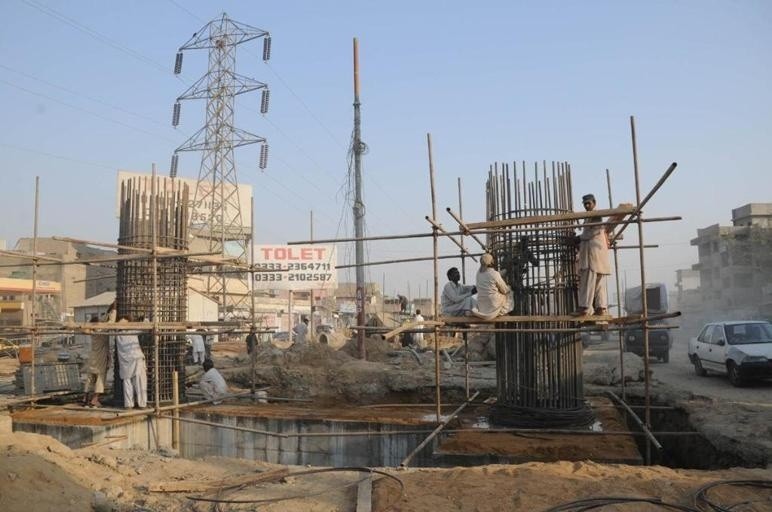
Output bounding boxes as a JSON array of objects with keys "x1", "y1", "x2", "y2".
[
  {"x1": 688, "y1": 318, "x2": 772, "y2": 387},
  {"x1": 581, "y1": 326, "x2": 609, "y2": 348}
]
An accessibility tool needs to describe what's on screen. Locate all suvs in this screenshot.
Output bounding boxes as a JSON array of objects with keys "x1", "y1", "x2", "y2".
[{"x1": 620, "y1": 315, "x2": 672, "y2": 365}]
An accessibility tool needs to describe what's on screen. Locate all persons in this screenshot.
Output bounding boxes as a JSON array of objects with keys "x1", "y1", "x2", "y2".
[
  {"x1": 410, "y1": 308, "x2": 428, "y2": 344},
  {"x1": 502, "y1": 239, "x2": 540, "y2": 300},
  {"x1": 198, "y1": 360, "x2": 227, "y2": 407},
  {"x1": 78, "y1": 302, "x2": 116, "y2": 408},
  {"x1": 243, "y1": 332, "x2": 258, "y2": 353},
  {"x1": 292, "y1": 318, "x2": 309, "y2": 346},
  {"x1": 112, "y1": 314, "x2": 151, "y2": 409},
  {"x1": 187, "y1": 333, "x2": 208, "y2": 364},
  {"x1": 395, "y1": 295, "x2": 409, "y2": 312},
  {"x1": 441, "y1": 267, "x2": 477, "y2": 317},
  {"x1": 573, "y1": 194, "x2": 610, "y2": 319},
  {"x1": 477, "y1": 253, "x2": 514, "y2": 319}
]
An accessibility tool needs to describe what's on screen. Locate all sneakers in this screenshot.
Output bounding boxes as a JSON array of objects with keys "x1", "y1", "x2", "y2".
[
  {"x1": 593, "y1": 307, "x2": 607, "y2": 315},
  {"x1": 570, "y1": 307, "x2": 589, "y2": 315}
]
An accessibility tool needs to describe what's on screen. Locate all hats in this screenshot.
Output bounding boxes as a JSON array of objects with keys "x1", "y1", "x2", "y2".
[
  {"x1": 581, "y1": 194, "x2": 594, "y2": 202},
  {"x1": 478, "y1": 254, "x2": 494, "y2": 267}
]
